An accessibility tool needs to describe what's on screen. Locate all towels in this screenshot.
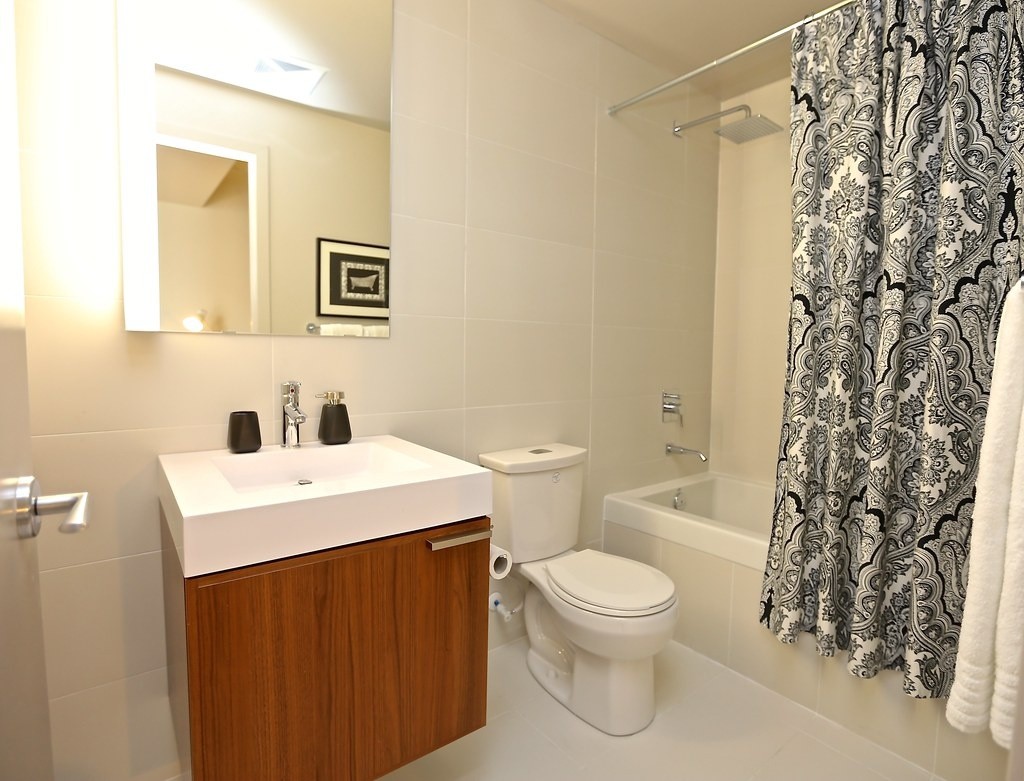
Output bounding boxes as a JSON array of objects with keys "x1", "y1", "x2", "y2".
[
  {"x1": 943, "y1": 273, "x2": 1023, "y2": 736},
  {"x1": 987, "y1": 391, "x2": 1023, "y2": 753},
  {"x1": 320, "y1": 321, "x2": 363, "y2": 337},
  {"x1": 361, "y1": 325, "x2": 390, "y2": 338}
]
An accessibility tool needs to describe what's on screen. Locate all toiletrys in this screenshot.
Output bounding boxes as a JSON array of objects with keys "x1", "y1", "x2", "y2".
[{"x1": 314, "y1": 388, "x2": 355, "y2": 445}]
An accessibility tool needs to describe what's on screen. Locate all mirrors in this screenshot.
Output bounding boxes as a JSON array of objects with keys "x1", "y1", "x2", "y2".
[{"x1": 110, "y1": 0, "x2": 402, "y2": 340}]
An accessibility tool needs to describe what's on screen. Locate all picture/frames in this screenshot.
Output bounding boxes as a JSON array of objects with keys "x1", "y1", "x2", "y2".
[{"x1": 317, "y1": 236, "x2": 389, "y2": 321}]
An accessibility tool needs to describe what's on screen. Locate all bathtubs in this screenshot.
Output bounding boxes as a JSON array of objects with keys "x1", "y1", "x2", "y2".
[{"x1": 599, "y1": 464, "x2": 1013, "y2": 781}]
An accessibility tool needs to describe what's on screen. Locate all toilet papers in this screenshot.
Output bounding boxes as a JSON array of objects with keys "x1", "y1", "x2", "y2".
[{"x1": 490, "y1": 543, "x2": 514, "y2": 581}]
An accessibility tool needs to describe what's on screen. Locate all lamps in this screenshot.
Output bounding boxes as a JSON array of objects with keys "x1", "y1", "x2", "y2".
[{"x1": 182, "y1": 302, "x2": 216, "y2": 333}]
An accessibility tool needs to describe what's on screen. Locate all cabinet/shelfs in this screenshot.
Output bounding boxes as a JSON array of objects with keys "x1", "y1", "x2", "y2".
[{"x1": 156, "y1": 495, "x2": 490, "y2": 781}]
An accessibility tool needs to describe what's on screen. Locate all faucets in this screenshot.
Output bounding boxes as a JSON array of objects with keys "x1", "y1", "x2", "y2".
[
  {"x1": 664, "y1": 445, "x2": 710, "y2": 462},
  {"x1": 279, "y1": 377, "x2": 308, "y2": 451}
]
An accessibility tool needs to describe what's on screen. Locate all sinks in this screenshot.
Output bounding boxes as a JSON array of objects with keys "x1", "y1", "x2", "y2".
[{"x1": 152, "y1": 434, "x2": 498, "y2": 581}]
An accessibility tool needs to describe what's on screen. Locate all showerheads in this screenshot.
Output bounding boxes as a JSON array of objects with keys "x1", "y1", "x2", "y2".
[{"x1": 712, "y1": 112, "x2": 788, "y2": 146}]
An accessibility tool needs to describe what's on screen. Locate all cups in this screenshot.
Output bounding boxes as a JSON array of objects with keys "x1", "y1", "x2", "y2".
[{"x1": 227, "y1": 411, "x2": 262, "y2": 454}]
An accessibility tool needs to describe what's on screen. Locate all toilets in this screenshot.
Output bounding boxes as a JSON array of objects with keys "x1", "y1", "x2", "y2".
[{"x1": 478, "y1": 439, "x2": 682, "y2": 739}]
